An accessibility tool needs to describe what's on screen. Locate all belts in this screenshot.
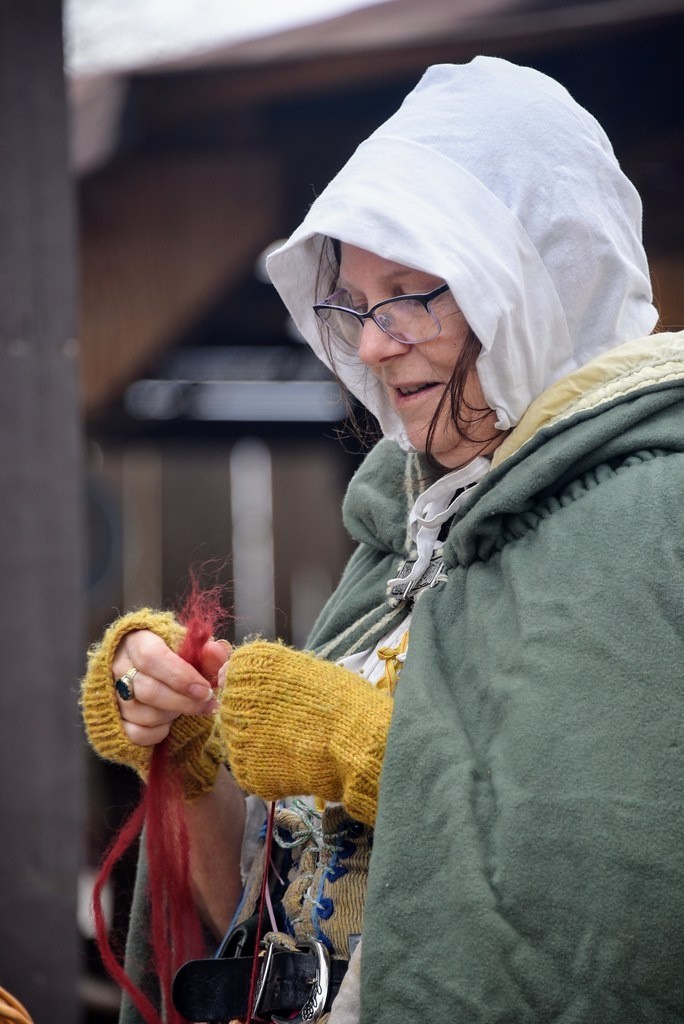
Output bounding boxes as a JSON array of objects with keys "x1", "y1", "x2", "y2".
[{"x1": 172, "y1": 940, "x2": 348, "y2": 1024}]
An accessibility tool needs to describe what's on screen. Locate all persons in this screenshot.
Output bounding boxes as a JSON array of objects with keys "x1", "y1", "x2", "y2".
[{"x1": 76, "y1": 55, "x2": 684, "y2": 1024}]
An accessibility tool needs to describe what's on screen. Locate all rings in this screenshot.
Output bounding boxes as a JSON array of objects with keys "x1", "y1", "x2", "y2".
[{"x1": 115, "y1": 666, "x2": 139, "y2": 700}]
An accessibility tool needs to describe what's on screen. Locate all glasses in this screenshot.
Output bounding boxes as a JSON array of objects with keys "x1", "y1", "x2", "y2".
[{"x1": 313, "y1": 283, "x2": 449, "y2": 349}]
